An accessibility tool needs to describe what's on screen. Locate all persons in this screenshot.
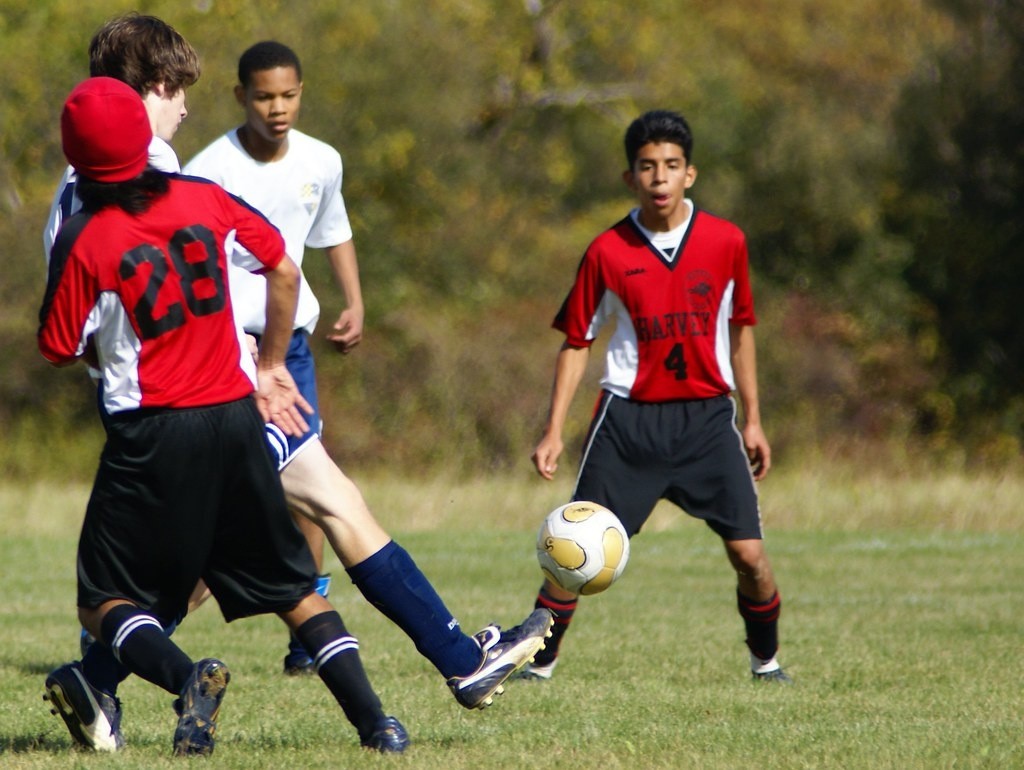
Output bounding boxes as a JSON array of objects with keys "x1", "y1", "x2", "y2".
[
  {"x1": 521, "y1": 109, "x2": 792, "y2": 683},
  {"x1": 43, "y1": 11, "x2": 554, "y2": 754},
  {"x1": 36, "y1": 77, "x2": 411, "y2": 757}
]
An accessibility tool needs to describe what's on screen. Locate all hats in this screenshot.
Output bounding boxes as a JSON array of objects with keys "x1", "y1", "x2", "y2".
[{"x1": 60, "y1": 76, "x2": 153, "y2": 182}]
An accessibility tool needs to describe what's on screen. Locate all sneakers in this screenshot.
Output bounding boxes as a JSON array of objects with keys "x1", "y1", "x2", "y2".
[
  {"x1": 751, "y1": 668, "x2": 792, "y2": 684},
  {"x1": 171, "y1": 657, "x2": 232, "y2": 759},
  {"x1": 497, "y1": 625, "x2": 560, "y2": 679},
  {"x1": 446, "y1": 607, "x2": 555, "y2": 711},
  {"x1": 40, "y1": 659, "x2": 126, "y2": 753},
  {"x1": 282, "y1": 655, "x2": 315, "y2": 676},
  {"x1": 358, "y1": 715, "x2": 411, "y2": 755}
]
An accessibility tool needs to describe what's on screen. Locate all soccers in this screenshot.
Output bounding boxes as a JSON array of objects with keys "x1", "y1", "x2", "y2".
[{"x1": 535, "y1": 500, "x2": 633, "y2": 598}]
